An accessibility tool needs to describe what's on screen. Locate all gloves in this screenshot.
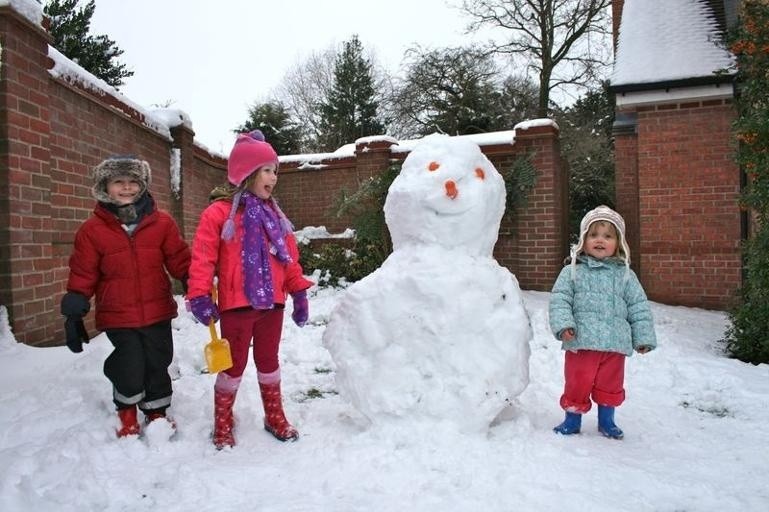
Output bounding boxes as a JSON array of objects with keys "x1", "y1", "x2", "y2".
[
  {"x1": 190, "y1": 294, "x2": 220, "y2": 325},
  {"x1": 61, "y1": 291, "x2": 89, "y2": 353},
  {"x1": 290, "y1": 289, "x2": 308, "y2": 327}
]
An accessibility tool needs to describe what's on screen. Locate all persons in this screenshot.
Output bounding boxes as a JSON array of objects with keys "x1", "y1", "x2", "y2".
[
  {"x1": 186, "y1": 130, "x2": 315, "y2": 449},
  {"x1": 548, "y1": 205, "x2": 656, "y2": 439},
  {"x1": 60, "y1": 155, "x2": 193, "y2": 439}
]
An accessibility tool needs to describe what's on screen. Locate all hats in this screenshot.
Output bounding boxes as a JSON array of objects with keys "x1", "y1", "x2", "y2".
[
  {"x1": 90, "y1": 154, "x2": 152, "y2": 206},
  {"x1": 572, "y1": 204, "x2": 631, "y2": 261},
  {"x1": 228, "y1": 129, "x2": 279, "y2": 187}
]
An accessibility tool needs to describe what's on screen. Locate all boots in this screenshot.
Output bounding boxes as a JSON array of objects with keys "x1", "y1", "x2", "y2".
[
  {"x1": 256, "y1": 364, "x2": 299, "y2": 442},
  {"x1": 214, "y1": 371, "x2": 243, "y2": 450},
  {"x1": 145, "y1": 413, "x2": 176, "y2": 437},
  {"x1": 117, "y1": 402, "x2": 140, "y2": 436},
  {"x1": 598, "y1": 404, "x2": 624, "y2": 439},
  {"x1": 554, "y1": 410, "x2": 581, "y2": 434}
]
